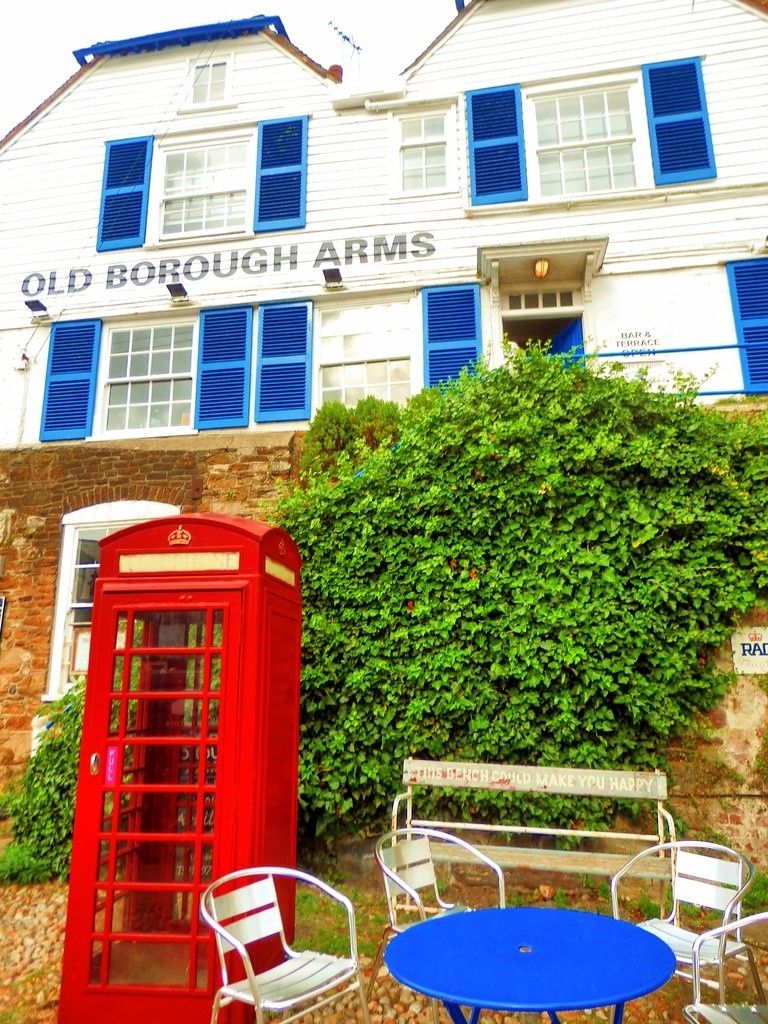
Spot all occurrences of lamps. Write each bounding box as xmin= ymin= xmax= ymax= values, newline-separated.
xmin=24 ymin=300 xmax=52 ymax=321
xmin=320 ymin=268 xmax=344 ymax=287
xmin=168 ymin=282 xmax=190 ymax=304
xmin=533 ymin=258 xmax=550 ymax=279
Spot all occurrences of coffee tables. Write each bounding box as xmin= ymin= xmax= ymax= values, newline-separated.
xmin=384 ymin=904 xmax=677 ymax=1024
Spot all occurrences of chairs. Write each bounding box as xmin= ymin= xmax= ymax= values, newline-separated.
xmin=611 ymin=841 xmax=766 ymax=1007
xmin=680 ymin=909 xmax=768 ymax=1024
xmin=360 ymin=827 xmax=506 ymax=1023
xmin=200 ymin=866 xmax=371 ymax=1024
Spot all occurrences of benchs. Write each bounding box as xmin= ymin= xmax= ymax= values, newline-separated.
xmin=388 ymin=758 xmax=680 ymax=927
